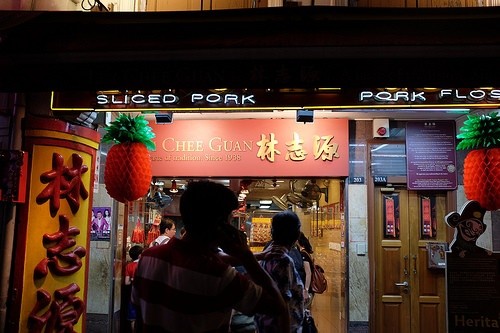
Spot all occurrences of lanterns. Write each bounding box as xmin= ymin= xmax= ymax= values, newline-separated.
xmin=99 ymin=112 xmax=157 ymax=204
xmin=455 ymin=112 xmax=499 ymax=209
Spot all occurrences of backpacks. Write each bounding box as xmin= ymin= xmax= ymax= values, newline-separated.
xmin=302 ymin=250 xmax=328 ymax=294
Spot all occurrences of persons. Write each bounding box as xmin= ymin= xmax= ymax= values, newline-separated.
xmin=253 ymin=209 xmax=319 ymax=333
xmin=216 ymin=245 xmax=285 ymax=274
xmin=125 ymin=245 xmax=143 ymax=331
xmin=179 ymin=227 xmax=187 ymax=239
xmin=91 ymin=208 xmax=112 ymax=240
xmin=148 ymin=217 xmax=177 ymax=248
xmin=130 ymin=178 xmax=286 ymax=333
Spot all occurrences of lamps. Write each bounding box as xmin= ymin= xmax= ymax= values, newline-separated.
xmin=169 ymin=179 xmax=178 ymax=193
xmin=155 ymin=112 xmax=173 ymax=125
xmin=296 ymin=110 xmax=314 ymax=124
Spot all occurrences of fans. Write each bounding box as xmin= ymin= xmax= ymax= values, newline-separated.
xmin=146 ymin=192 xmax=172 ymax=209
xmin=271 ymin=179 xmax=328 ymax=212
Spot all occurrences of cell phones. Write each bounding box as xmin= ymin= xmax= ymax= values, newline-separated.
xmin=218 ymin=223 xmax=246 ymax=247
